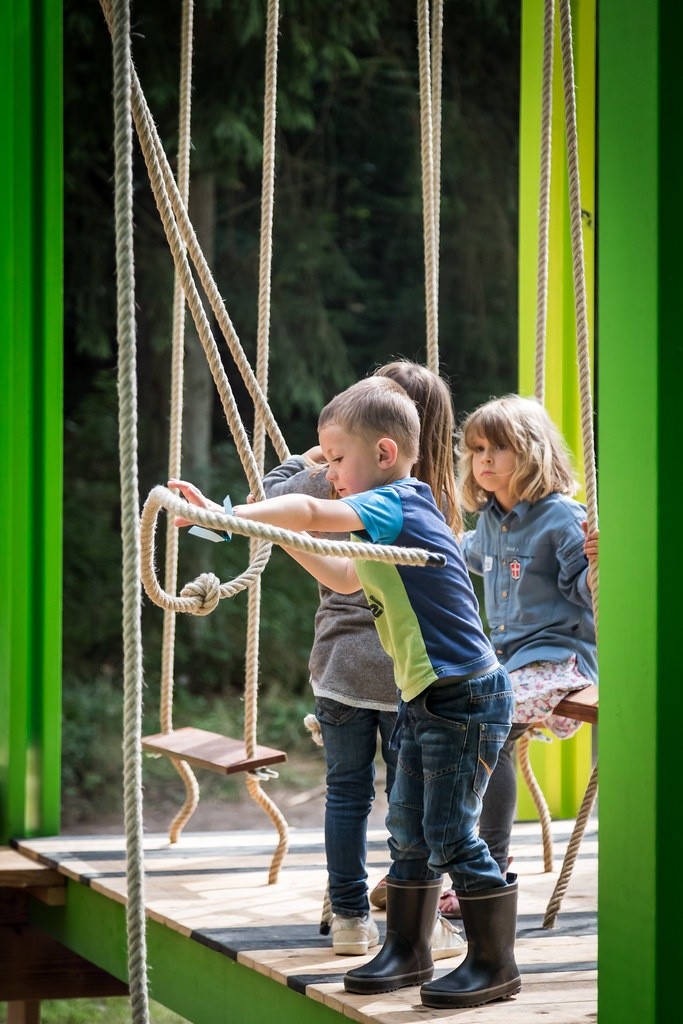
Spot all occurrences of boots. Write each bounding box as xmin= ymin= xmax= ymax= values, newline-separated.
xmin=344 ymin=874 xmax=444 ymax=994
xmin=420 ymin=872 xmax=521 ymax=1008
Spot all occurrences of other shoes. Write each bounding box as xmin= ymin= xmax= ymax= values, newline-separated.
xmin=370 ymin=877 xmax=387 ymax=908
xmin=437 ymin=889 xmax=463 ymax=919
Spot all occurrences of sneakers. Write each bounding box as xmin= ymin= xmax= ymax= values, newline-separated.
xmin=430 ymin=915 xmax=464 ymax=961
xmin=331 ymin=911 xmax=379 ymax=955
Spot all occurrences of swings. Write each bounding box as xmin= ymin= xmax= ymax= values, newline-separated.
xmin=103 ymin=0 xmax=599 ymax=1024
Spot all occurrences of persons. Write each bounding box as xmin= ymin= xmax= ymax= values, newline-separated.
xmin=261 ymin=361 xmax=464 ymax=961
xmin=167 ymin=377 xmax=521 ymax=1009
xmin=436 ymin=395 xmax=599 ymax=921
